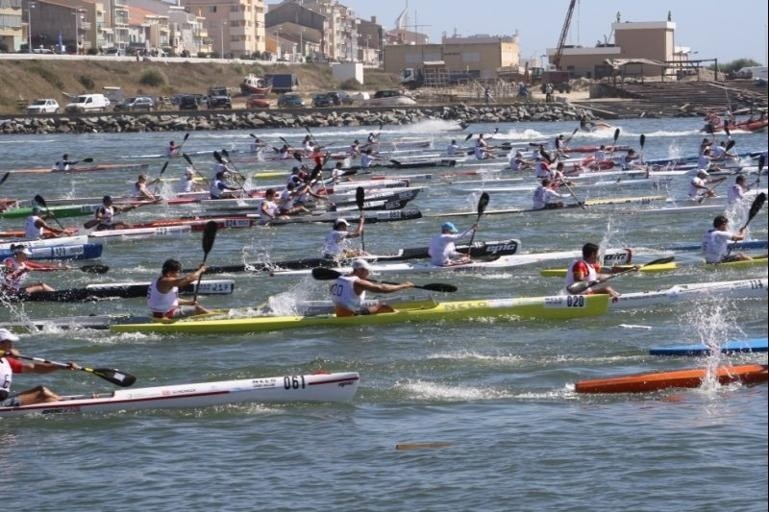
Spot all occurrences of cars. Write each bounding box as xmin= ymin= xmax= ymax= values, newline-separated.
xmin=28 ymin=42 xmax=169 ymax=59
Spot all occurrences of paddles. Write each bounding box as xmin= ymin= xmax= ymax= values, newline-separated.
xmin=6 ymin=351 xmax=136 ymax=386
xmin=695 ymin=126 xmax=768 ymax=260
xmin=355 ymin=184 xmax=366 ymax=251
xmin=454 ymin=126 xmax=644 ymax=166
xmin=193 ymin=221 xmax=218 ymax=300
xmin=312 ymin=268 xmax=457 ymax=292
xmin=85 ymin=206 xmax=132 ymax=229
xmin=568 ymin=256 xmax=675 ymax=295
xmin=152 ymin=125 xmax=400 ymax=197
xmin=34 ymin=195 xmax=65 ymax=234
xmin=71 ymin=157 xmax=95 ymax=164
xmin=24 ymin=263 xmax=108 ymax=274
xmin=468 ymin=192 xmax=489 ymax=255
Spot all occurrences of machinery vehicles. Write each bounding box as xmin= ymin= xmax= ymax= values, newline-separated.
xmin=540 ymin=0 xmax=581 ymax=93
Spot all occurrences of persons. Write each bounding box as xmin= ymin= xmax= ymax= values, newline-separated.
xmin=561 ymin=242 xmax=622 ymax=300
xmin=494 ymin=128 xmax=499 ymax=134
xmin=510 ymin=133 xmax=642 ymax=209
xmin=128 ymin=135 xmax=383 ymax=221
xmin=145 ymin=216 xmax=481 ymax=319
xmin=447 ymin=133 xmax=495 ymax=160
xmin=2 ymin=153 xmax=138 ymax=407
xmin=688 ymin=109 xmax=754 ymax=267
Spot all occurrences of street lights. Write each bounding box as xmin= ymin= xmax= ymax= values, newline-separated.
xmin=365 ymin=31 xmax=373 ymax=63
xmin=27 ymin=1 xmax=36 ymax=53
xmin=219 ymin=20 xmax=227 ymax=58
xmin=74 ymin=5 xmax=83 ymax=54
xmin=299 ymin=27 xmax=305 ymax=57
xmin=276 ymin=25 xmax=282 ymax=49
xmin=117 ymin=8 xmax=125 ymax=49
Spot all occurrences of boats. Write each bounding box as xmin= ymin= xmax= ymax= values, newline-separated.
xmin=645 ymin=338 xmax=769 ymax=356
xmin=0 ymin=369 xmax=361 ymax=424
xmin=239 ymin=72 xmax=273 ymax=96
xmin=574 ymin=359 xmax=769 ymax=394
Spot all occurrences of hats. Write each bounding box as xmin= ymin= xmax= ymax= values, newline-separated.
xmin=0 ymin=328 xmax=20 ymax=343
xmin=13 ymin=247 xmax=32 ymax=255
xmin=352 ymin=259 xmax=374 ymax=273
xmin=697 ymin=168 xmax=710 ymax=176
xmin=441 ymin=221 xmax=459 ymax=234
xmin=335 ymin=217 xmax=351 ymax=227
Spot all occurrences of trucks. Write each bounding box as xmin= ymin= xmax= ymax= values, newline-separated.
xmin=401 ymin=65 xmax=480 ymax=89
xmin=256 ymin=73 xmax=299 ymax=94
xmin=495 ymin=64 xmax=544 ymax=85
